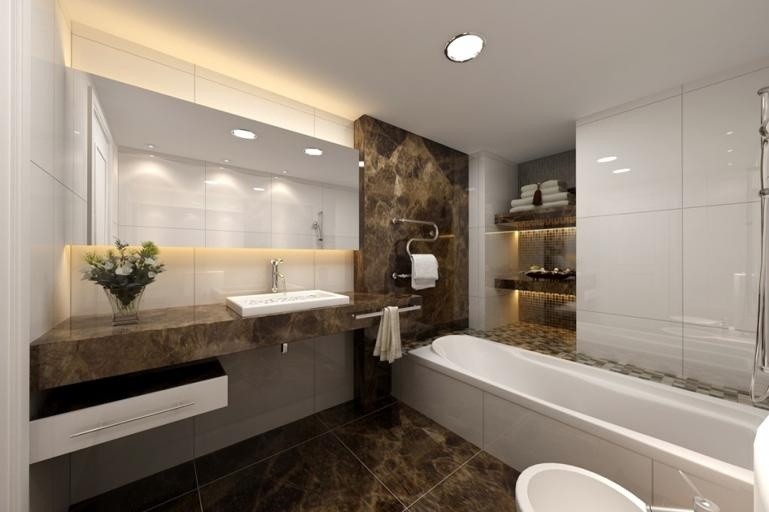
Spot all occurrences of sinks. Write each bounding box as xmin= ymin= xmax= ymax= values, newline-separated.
xmin=517 ymin=462 xmax=649 ymax=512
xmin=226 ymin=290 xmax=350 ymax=319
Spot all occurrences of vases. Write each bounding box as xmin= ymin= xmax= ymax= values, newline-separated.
xmin=107 ymin=285 xmax=143 ymax=323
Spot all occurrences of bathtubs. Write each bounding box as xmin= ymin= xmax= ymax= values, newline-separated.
xmin=410 ymin=332 xmax=768 ymax=497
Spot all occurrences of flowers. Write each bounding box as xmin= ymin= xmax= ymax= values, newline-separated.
xmin=78 ymin=244 xmax=161 ymax=315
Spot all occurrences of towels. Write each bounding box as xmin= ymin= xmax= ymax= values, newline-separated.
xmin=407 ymin=254 xmax=437 ymax=290
xmin=373 ymin=306 xmax=406 ymax=364
xmin=509 ymin=180 xmax=575 ymax=215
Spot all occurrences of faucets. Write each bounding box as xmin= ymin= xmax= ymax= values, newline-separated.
xmin=272 ymin=258 xmax=284 ymax=291
xmin=646 ymin=468 xmax=721 ymax=512
